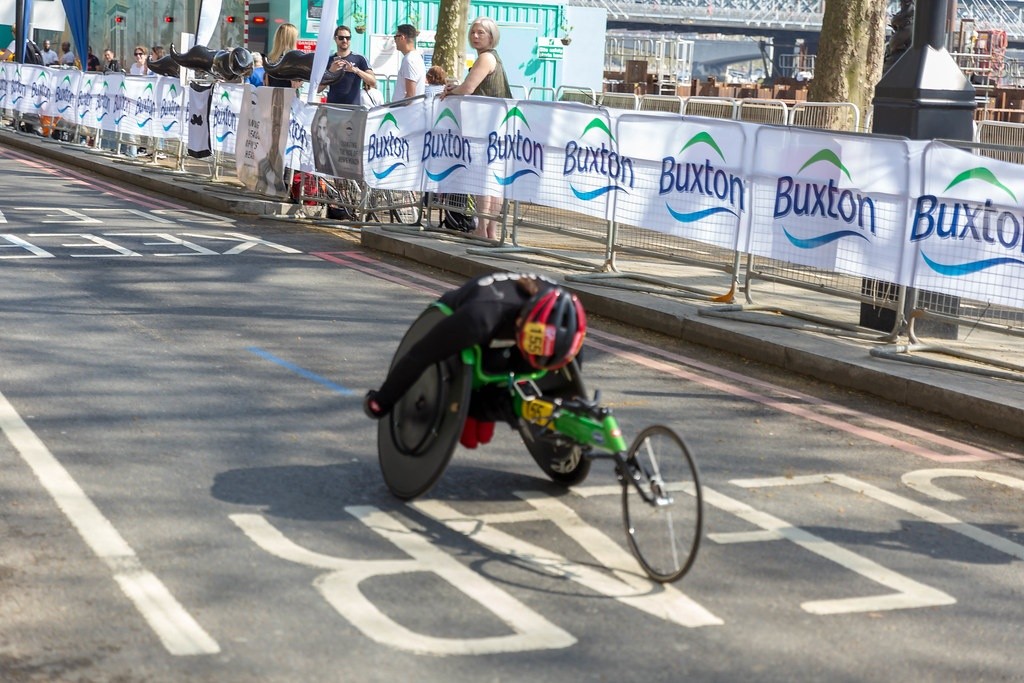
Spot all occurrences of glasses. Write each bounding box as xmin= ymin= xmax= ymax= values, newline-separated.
xmin=134 ymin=53 xmax=143 ymax=56
xmin=336 ymin=36 xmax=351 ymax=40
xmin=394 ymin=35 xmax=406 ymax=40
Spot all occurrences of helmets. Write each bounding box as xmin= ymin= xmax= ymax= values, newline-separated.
xmin=515 ymin=286 xmax=587 ymax=371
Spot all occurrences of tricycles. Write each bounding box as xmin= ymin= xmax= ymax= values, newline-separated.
xmin=377 ymin=297 xmax=704 ymax=585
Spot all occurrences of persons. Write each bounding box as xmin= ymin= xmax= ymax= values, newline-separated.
xmin=439 ymin=17 xmax=513 ymax=236
xmin=317 ymin=25 xmax=377 ymax=104
xmin=244 ymin=51 xmax=266 ymax=86
xmin=265 ymin=23 xmax=304 ymax=97
xmin=361 ymin=80 xmax=384 ymax=109
xmin=60 ymin=41 xmax=74 ymax=66
xmin=40 ymin=39 xmax=59 ymax=66
xmin=144 ymin=46 xmax=166 ymax=159
xmin=0 ymin=23 xmax=36 ymax=63
xmin=102 ymin=48 xmax=121 ymax=72
xmin=385 ymin=25 xmax=426 ymax=213
xmin=87 ymin=47 xmax=100 ymax=71
xmin=425 ymin=66 xmax=447 ymax=97
xmin=362 ymin=272 xmax=588 ymax=449
xmin=129 ymin=45 xmax=150 ymax=155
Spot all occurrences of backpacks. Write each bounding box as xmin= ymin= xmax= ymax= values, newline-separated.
xmin=26 ymin=40 xmax=44 ymax=66
xmin=438 ymin=193 xmax=471 ymax=233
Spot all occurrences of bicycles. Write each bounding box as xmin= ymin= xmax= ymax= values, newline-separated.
xmin=280 ymin=166 xmax=482 ymax=230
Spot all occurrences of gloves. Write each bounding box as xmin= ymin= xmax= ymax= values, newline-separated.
xmin=363 ymin=389 xmax=389 ymax=419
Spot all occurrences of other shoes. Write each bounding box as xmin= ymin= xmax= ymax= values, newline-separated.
xmin=478 ymin=421 xmax=494 ymax=444
xmin=384 ymin=200 xmax=418 ymax=215
xmin=469 ymin=229 xmax=496 ymax=241
xmin=459 ymin=417 xmax=479 ymax=448
xmin=137 ymin=147 xmax=148 ymax=157
xmin=146 ymin=151 xmax=167 ymax=160
